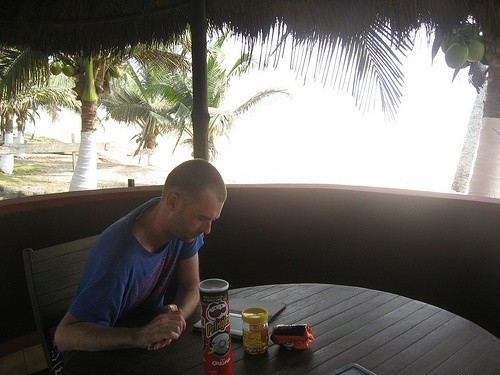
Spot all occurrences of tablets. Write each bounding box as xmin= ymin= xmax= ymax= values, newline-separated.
xmin=193 ymin=313 xmax=243 ymax=339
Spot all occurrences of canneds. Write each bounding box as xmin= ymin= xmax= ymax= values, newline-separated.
xmin=242 ymin=307 xmax=269 ymax=357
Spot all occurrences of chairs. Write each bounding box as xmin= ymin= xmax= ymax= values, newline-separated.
xmin=23 ymin=235 xmax=101 ymax=375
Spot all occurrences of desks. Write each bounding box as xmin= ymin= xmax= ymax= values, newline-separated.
xmin=64 ymin=283 xmax=500 ymax=375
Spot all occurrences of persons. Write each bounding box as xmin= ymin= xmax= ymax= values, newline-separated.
xmin=49 ymin=159 xmax=228 ymax=375
